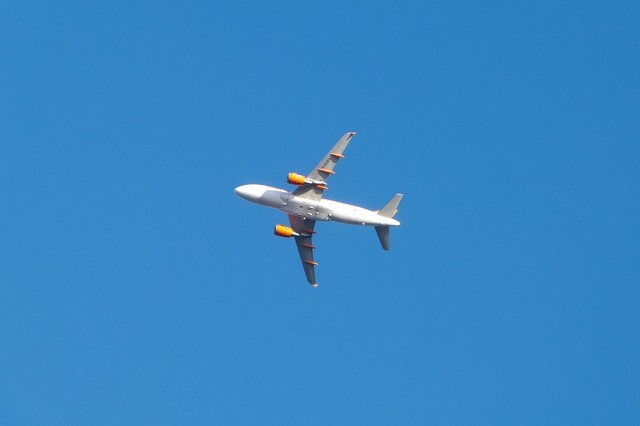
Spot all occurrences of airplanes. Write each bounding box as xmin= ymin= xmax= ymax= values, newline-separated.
xmin=234 ymin=132 xmax=403 ymax=287
